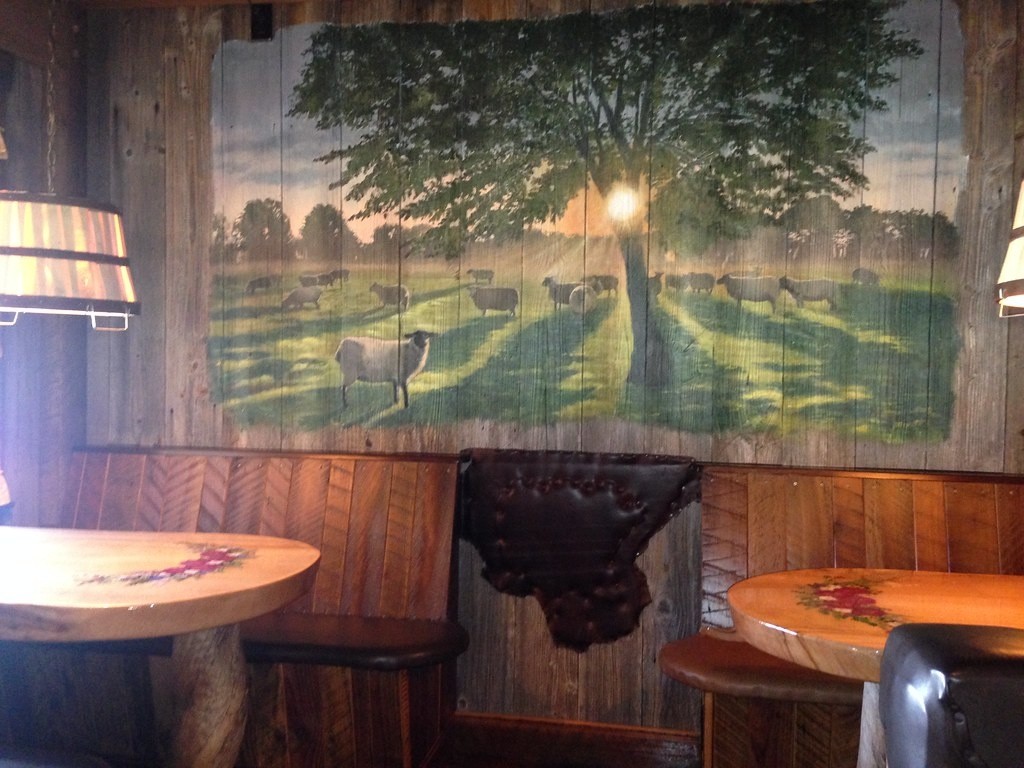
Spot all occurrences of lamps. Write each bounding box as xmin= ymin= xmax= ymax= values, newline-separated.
xmin=1 ymin=189 xmax=140 ymax=331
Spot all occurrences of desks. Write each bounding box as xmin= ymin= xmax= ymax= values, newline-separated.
xmin=0 ymin=525 xmax=321 ymax=768
xmin=724 ymin=566 xmax=1024 ymax=768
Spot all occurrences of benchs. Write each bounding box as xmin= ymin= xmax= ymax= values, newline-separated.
xmin=19 ymin=446 xmax=1024 ymax=768
